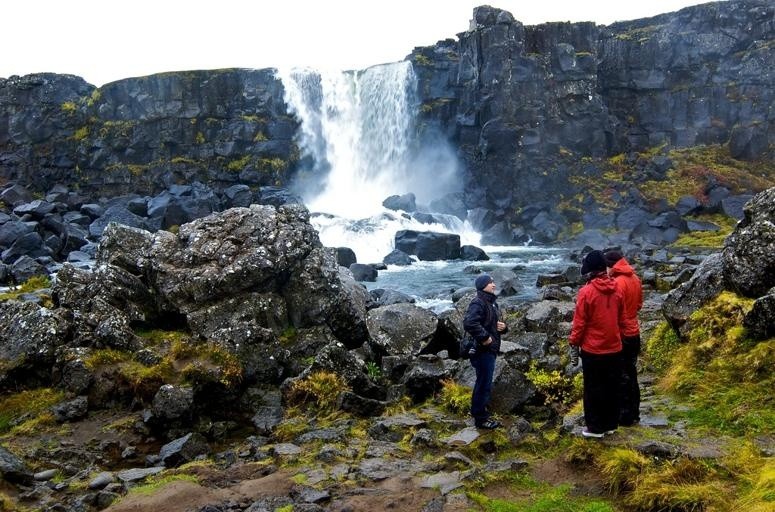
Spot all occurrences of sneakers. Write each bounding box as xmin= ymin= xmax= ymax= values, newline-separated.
xmin=606 ymin=427 xmax=620 ymax=435
xmin=581 ymin=428 xmax=606 ymax=439
xmin=474 ymin=419 xmax=501 ymax=430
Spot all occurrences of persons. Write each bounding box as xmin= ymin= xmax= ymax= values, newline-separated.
xmin=566 ymin=247 xmax=624 ymax=440
xmin=464 ymin=274 xmax=509 ymax=430
xmin=603 ymin=252 xmax=644 ymax=423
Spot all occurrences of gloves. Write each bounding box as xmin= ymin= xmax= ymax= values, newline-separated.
xmin=569 ymin=344 xmax=579 ymax=367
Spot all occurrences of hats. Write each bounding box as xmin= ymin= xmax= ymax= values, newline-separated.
xmin=604 ymin=249 xmax=624 ymax=269
xmin=474 ymin=275 xmax=495 ymax=291
xmin=579 ymin=249 xmax=607 ymax=275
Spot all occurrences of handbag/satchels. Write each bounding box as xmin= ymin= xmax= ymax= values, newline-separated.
xmin=459 ymin=327 xmax=481 ymax=361
